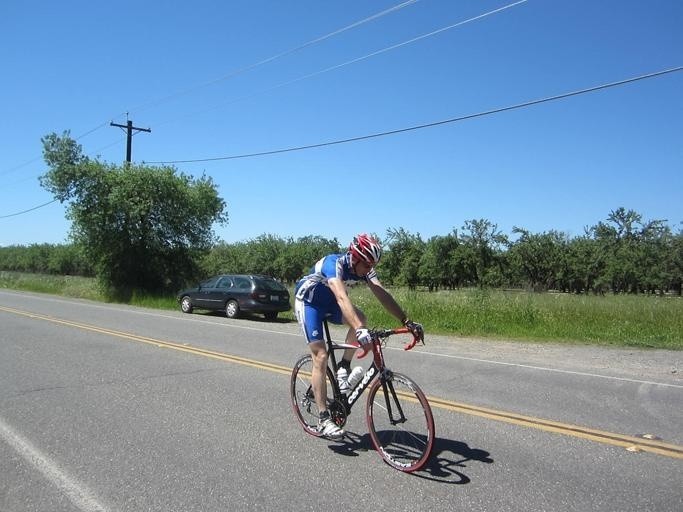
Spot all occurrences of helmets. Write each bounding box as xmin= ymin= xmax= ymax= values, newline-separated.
xmin=348 ymin=234 xmax=382 ymax=263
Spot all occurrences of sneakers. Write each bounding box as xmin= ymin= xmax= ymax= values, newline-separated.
xmin=316 ymin=416 xmax=346 ymax=437
xmin=334 ymin=364 xmax=352 ymax=382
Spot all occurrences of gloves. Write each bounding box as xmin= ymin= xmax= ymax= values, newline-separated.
xmin=356 ymin=328 xmax=373 ymax=345
xmin=404 ymin=320 xmax=424 ymax=339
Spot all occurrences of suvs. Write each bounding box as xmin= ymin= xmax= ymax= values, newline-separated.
xmin=174 ymin=271 xmax=291 ymax=321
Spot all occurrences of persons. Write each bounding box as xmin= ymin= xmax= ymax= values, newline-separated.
xmin=293 ymin=231 xmax=426 ymax=440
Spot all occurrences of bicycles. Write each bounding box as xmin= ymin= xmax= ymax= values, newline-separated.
xmin=286 ymin=305 xmax=437 ymax=477
